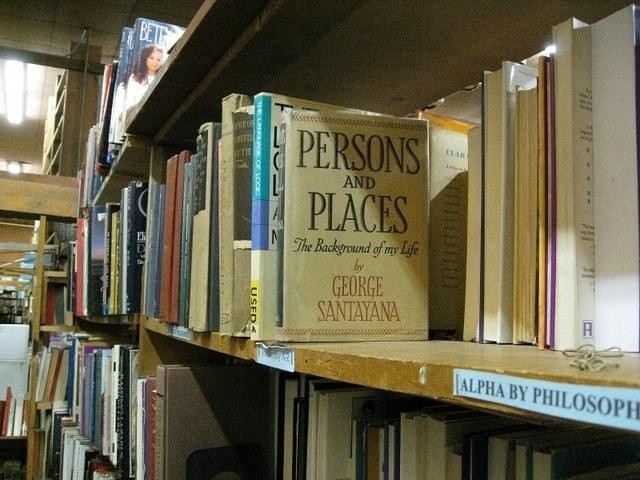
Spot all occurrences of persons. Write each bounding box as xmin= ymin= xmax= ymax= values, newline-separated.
xmin=129 ymin=44 xmax=164 ymax=105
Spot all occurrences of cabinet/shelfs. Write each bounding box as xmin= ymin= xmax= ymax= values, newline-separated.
xmin=42 ymin=43 xmax=102 ymax=177
xmin=0 ymin=290 xmax=31 ymax=323
xmin=65 ymin=135 xmax=152 ymax=326
xmin=125 ymin=0 xmax=640 ymax=432
xmin=27 ymin=216 xmax=79 ymax=478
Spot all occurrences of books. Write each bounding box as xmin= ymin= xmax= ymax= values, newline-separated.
xmin=80 ymin=16 xmax=188 ymax=209
xmin=134 ymin=359 xmax=636 ymax=480
xmin=144 ymin=7 xmax=637 ymax=344
xmin=73 ymin=179 xmax=148 ymax=314
xmin=0 ymin=332 xmax=139 ymax=480
